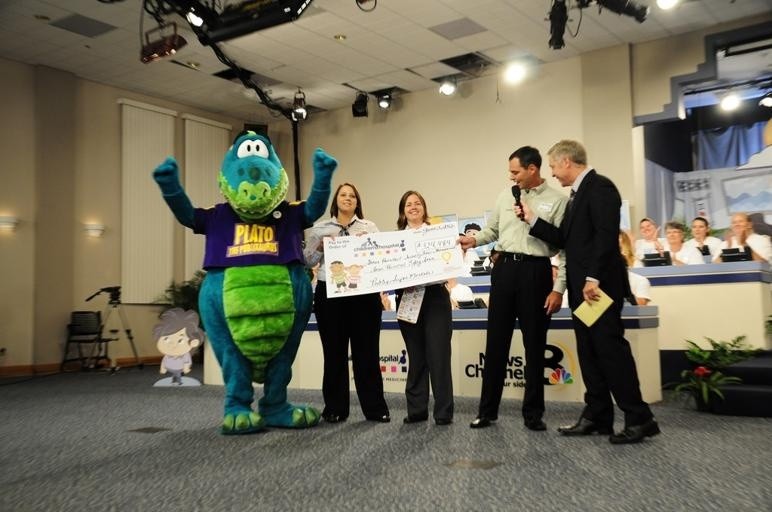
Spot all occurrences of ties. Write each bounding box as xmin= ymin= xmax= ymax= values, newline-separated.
xmin=569 ymin=189 xmax=577 ymax=210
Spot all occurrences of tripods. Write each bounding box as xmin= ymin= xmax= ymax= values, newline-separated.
xmin=85 ymin=286 xmax=141 ymax=369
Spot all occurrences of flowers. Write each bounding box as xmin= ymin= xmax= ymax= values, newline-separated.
xmin=669 ymin=367 xmax=742 ymax=403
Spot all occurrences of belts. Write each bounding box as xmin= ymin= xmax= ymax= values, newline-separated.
xmin=502 ymin=253 xmax=534 ymax=261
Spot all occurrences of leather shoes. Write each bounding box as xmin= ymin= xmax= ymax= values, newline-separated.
xmin=366 ymin=414 xmax=391 ymax=423
xmin=470 ymin=417 xmax=498 ymax=429
xmin=325 ymin=414 xmax=340 ymax=423
xmin=558 ymin=418 xmax=615 ymax=436
xmin=436 ymin=420 xmax=451 ymax=425
xmin=524 ymin=420 xmax=547 ymax=431
xmin=609 ymin=417 xmax=662 ymax=445
xmin=403 ymin=417 xmax=415 ymax=424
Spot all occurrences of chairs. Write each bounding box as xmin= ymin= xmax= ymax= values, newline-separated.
xmin=61 ymin=311 xmax=118 ymax=373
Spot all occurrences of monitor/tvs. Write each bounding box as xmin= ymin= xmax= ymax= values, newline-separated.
xmin=189 ymin=0 xmax=339 ymax=45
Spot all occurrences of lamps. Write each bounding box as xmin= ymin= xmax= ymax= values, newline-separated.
xmin=291 ymin=97 xmax=308 ymax=122
xmin=758 ymin=90 xmax=772 ymax=110
xmin=378 ymin=91 xmax=392 ymax=110
xmin=440 ymin=74 xmax=457 ymax=98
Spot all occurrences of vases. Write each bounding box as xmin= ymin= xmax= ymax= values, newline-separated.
xmin=694 ymin=394 xmax=710 ymax=412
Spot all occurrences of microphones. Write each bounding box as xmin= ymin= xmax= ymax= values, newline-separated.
xmin=512 ymin=185 xmax=524 ymax=221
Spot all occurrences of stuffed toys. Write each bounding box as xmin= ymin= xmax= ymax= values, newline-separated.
xmin=150 ymin=127 xmax=339 ymax=438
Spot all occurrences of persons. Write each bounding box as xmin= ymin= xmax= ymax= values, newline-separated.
xmin=618 ymin=212 xmax=771 ymax=305
xmin=300 ymin=181 xmax=393 ymax=425
xmin=454 ymin=144 xmax=567 ymax=434
xmin=447 ymin=277 xmax=476 ymax=308
xmin=390 ymin=188 xmax=455 ymax=429
xmin=513 ymin=139 xmax=663 ymax=447
